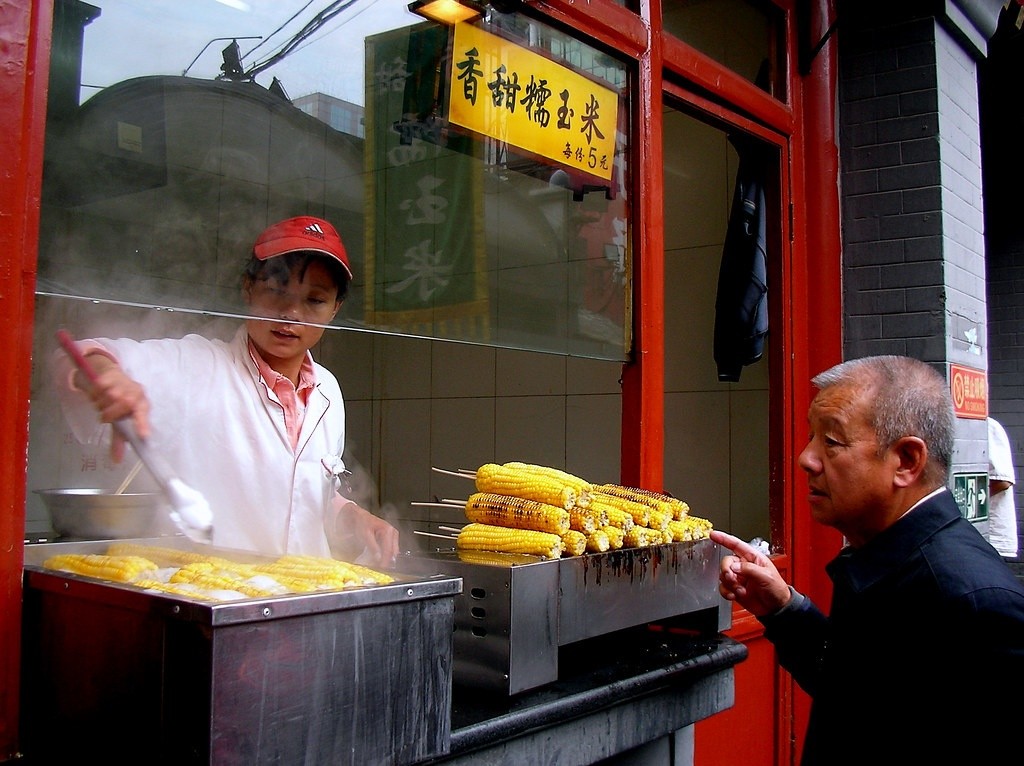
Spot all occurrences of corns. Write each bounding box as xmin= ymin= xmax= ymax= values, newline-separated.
xmin=42 ymin=543 xmax=396 ymax=604
xmin=455 ymin=550 xmax=542 ymax=566
xmin=455 ymin=461 xmax=712 ymax=560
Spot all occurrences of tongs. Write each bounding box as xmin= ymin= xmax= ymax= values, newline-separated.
xmin=54 ymin=329 xmax=215 ymax=544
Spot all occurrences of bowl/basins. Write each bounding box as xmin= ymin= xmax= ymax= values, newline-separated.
xmin=31 ymin=489 xmax=168 ymax=538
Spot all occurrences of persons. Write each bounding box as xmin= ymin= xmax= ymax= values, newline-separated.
xmin=986 ymin=415 xmax=1019 ymax=559
xmin=709 ymin=353 xmax=1024 ymax=766
xmin=45 ymin=214 xmax=401 ymax=568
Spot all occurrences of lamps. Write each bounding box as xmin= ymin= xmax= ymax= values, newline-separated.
xmin=406 ymin=0 xmax=487 ymax=26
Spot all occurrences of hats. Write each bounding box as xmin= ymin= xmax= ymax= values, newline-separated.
xmin=252 ymin=216 xmax=352 ymax=280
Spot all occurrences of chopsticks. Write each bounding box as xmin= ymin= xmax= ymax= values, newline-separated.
xmin=116 ymin=460 xmax=143 ymax=495
